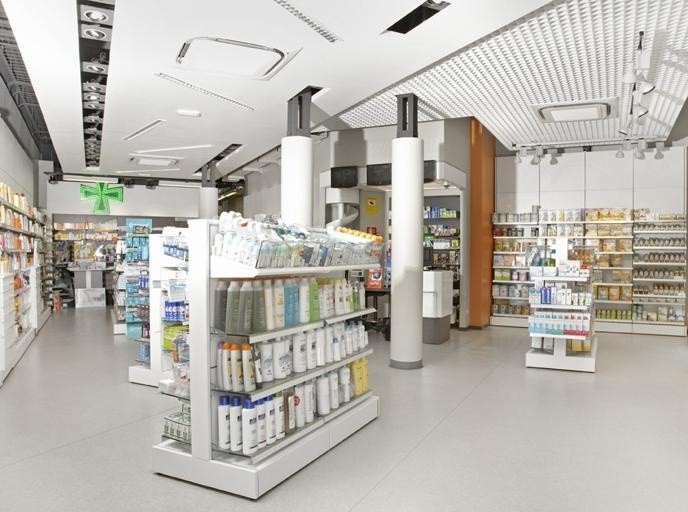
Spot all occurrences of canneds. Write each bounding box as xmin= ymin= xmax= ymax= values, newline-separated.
xmin=493 ymin=227 xmax=538 ymax=236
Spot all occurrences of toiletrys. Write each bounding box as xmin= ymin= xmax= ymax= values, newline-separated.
xmin=214 ymin=277 xmax=368 ymax=393
xmin=527 ymin=311 xmax=591 ymax=338
xmin=528 ymin=286 xmax=557 ymax=305
xmin=316 ymin=356 xmax=369 ymax=416
xmin=557 ymin=289 xmax=592 ymax=306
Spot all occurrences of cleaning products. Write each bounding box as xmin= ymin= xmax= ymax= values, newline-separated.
xmin=217 ymin=379 xmax=316 ymax=456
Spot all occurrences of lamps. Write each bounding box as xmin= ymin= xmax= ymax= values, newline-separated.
xmin=508 ymin=135 xmax=668 ymax=167
xmin=618 ymin=29 xmax=655 ymax=142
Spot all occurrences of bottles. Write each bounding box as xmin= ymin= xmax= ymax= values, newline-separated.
xmin=594 ymin=225 xmax=686 ymax=323
xmin=214 ymin=272 xmax=369 ymax=436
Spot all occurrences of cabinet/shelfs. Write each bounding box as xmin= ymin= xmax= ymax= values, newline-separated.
xmin=2 ymin=178 xmax=105 ymax=395
xmin=492 ymin=205 xmax=688 ymax=338
xmin=104 ymin=215 xmax=188 ymax=387
xmin=152 ymin=215 xmax=386 ymax=503
xmin=524 ymin=238 xmax=598 ymax=373
xmin=424 ymin=194 xmax=471 ymax=331
